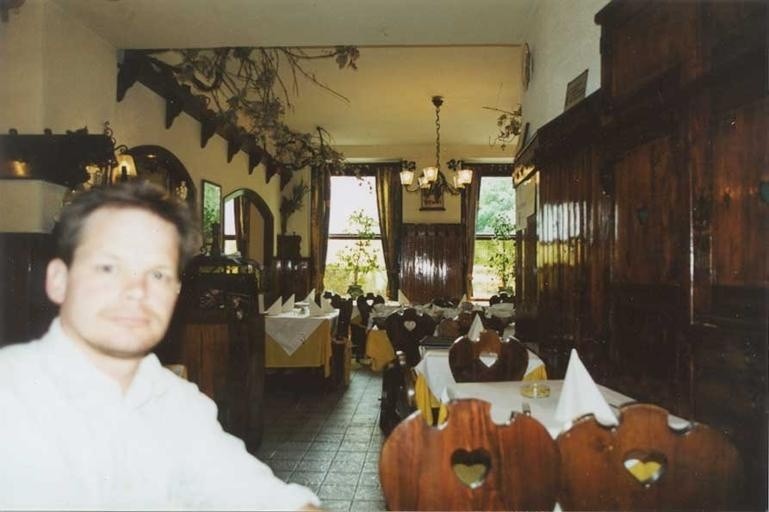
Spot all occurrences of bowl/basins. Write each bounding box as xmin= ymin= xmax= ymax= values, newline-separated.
xmin=1 ymin=160 xmax=32 ymax=178
xmin=520 ymin=384 xmax=551 ymax=397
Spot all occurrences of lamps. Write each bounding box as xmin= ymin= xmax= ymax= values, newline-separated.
xmin=63 ymin=119 xmax=138 ymax=205
xmin=398 ymin=95 xmax=473 ymax=201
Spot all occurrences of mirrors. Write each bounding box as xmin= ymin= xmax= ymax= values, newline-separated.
xmin=200 ymin=180 xmax=223 ymax=254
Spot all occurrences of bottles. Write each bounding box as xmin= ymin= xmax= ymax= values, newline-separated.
xmin=175 ymin=180 xmax=189 ymax=201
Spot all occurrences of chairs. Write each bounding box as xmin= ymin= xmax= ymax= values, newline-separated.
xmin=314 ymin=292 xmax=521 ymax=384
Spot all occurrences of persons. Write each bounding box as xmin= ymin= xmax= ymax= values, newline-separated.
xmin=0 ymin=173 xmax=332 ymax=511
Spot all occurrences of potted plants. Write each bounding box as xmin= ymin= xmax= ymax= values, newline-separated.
xmin=335 ymin=207 xmax=380 ymax=298
xmin=485 ymin=212 xmax=517 ymax=295
xmin=276 ymin=175 xmax=311 ymax=266
